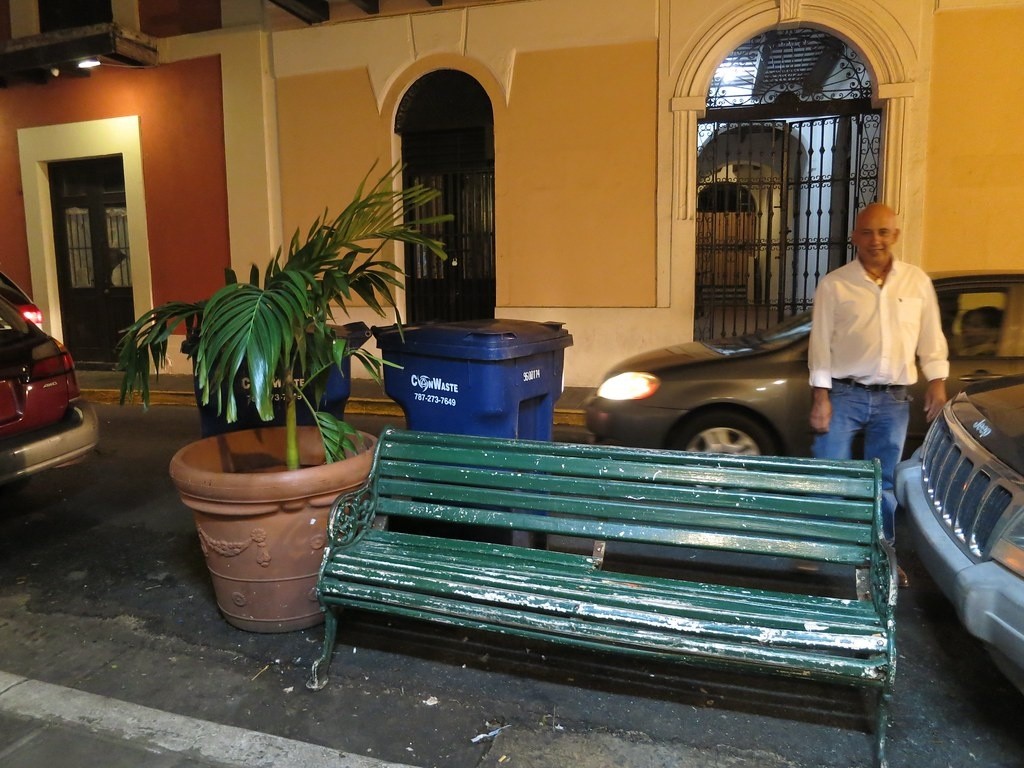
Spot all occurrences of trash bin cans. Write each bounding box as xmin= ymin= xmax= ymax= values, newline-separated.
xmin=180 ymin=319 xmax=376 ymax=434
xmin=372 ymin=320 xmax=574 ymax=550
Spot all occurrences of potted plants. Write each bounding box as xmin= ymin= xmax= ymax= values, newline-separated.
xmin=108 ymin=156 xmax=454 ymax=633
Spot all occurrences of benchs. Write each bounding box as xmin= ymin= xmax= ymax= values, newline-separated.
xmin=308 ymin=426 xmax=897 ymax=768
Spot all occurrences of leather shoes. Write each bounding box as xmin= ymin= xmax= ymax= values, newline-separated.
xmin=896 ymin=566 xmax=910 ymax=589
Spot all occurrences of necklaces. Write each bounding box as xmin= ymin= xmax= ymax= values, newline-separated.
xmin=865 ymin=259 xmax=890 ymax=285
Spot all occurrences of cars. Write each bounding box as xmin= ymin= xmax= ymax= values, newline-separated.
xmin=585 ymin=266 xmax=1024 ymax=492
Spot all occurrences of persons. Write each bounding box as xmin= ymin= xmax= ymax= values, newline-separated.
xmin=807 ymin=204 xmax=949 ymax=601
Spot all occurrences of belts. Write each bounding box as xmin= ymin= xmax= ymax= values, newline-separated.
xmin=831 ymin=377 xmax=905 ymax=392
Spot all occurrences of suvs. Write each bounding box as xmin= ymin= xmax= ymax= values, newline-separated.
xmin=893 ymin=373 xmax=1024 ymax=694
xmin=0 ymin=272 xmax=99 ymax=486
